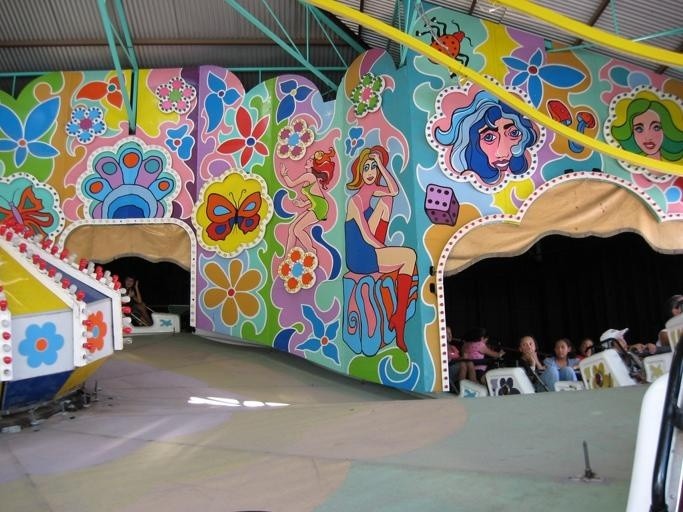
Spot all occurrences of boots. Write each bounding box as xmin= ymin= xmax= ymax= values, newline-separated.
xmin=388 ymin=273 xmax=412 ymax=352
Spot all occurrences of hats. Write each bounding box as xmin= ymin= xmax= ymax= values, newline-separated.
xmin=600 ymin=327 xmax=629 ymax=348
xmin=667 ymin=295 xmax=683 ymax=307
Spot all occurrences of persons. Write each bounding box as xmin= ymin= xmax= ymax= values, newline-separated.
xmin=444 ymin=320 xmax=664 ymax=394
xmin=120 ymin=273 xmax=153 ymax=326
xmin=665 ymin=293 xmax=682 ymax=330
